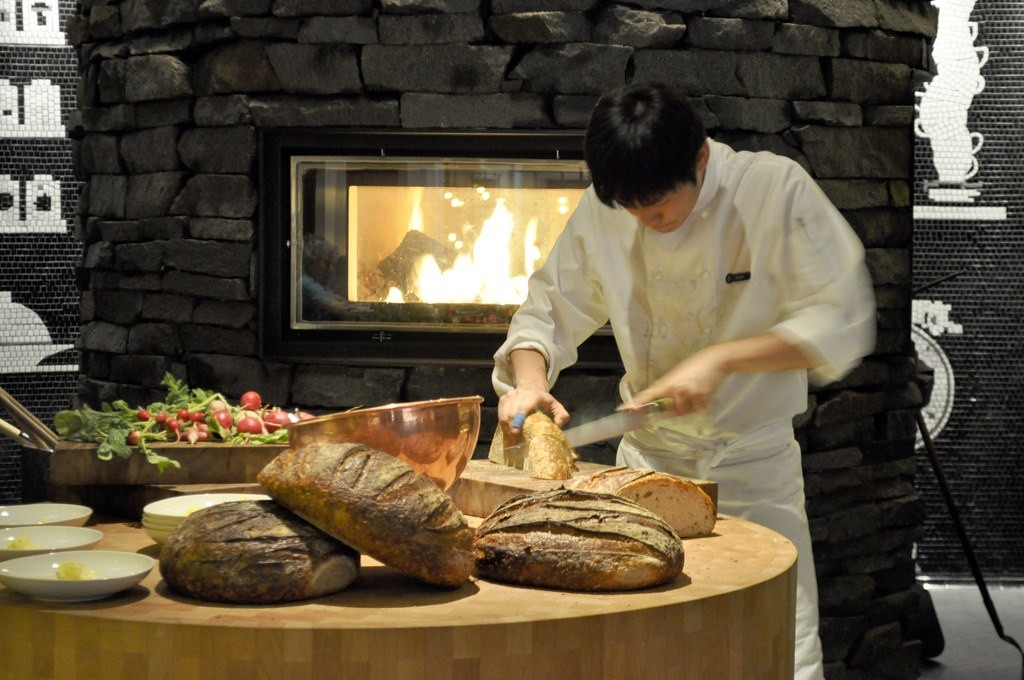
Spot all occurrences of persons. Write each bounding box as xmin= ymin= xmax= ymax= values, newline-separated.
xmin=485 ymin=80 xmax=877 ymax=680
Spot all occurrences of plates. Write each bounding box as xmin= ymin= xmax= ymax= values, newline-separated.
xmin=1 ymin=551 xmax=154 ymax=603
xmin=1 ymin=526 xmax=103 ymax=561
xmin=141 ymin=493 xmax=275 ymax=549
xmin=1 ymin=502 xmax=93 ymax=531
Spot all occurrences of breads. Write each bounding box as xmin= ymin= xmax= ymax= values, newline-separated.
xmin=160 ymin=501 xmax=362 ymax=602
xmin=258 ymin=442 xmax=476 ymax=588
xmin=490 ymin=411 xmax=578 ymax=480
xmin=572 ymin=467 xmax=717 ymax=536
xmin=472 ymin=490 xmax=684 ymax=591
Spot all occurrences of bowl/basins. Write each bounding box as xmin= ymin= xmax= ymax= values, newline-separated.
xmin=281 ymin=395 xmax=485 ymax=492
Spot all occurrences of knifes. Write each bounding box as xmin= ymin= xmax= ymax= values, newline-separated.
xmin=500 ymin=397 xmax=682 ymax=457
